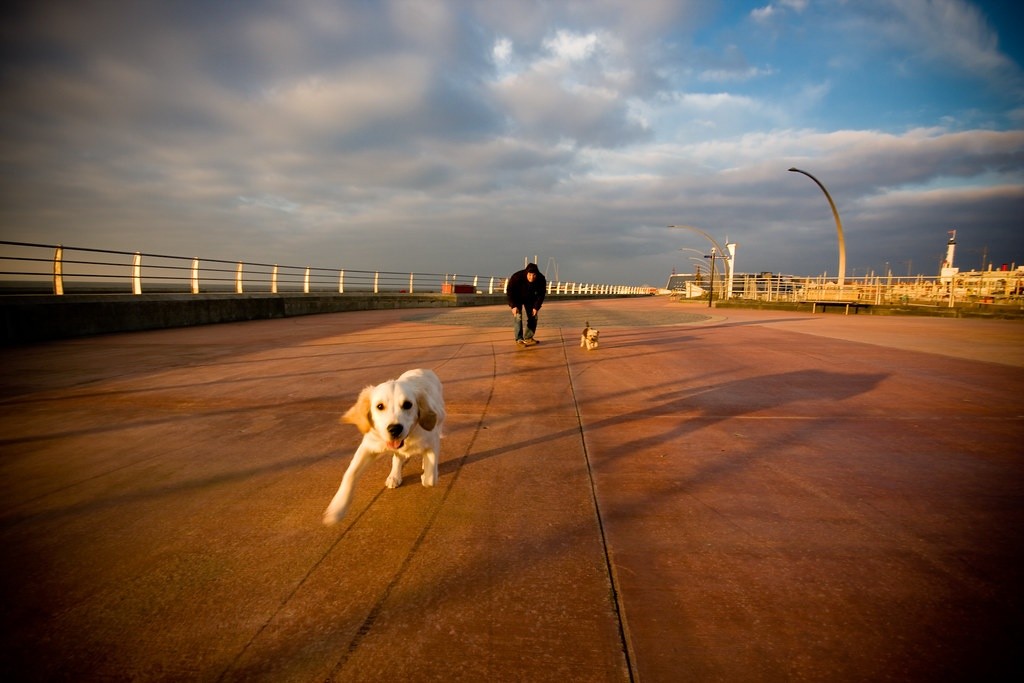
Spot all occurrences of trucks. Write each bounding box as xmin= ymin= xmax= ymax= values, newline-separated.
xmin=440 ymin=282 xmax=483 ymax=296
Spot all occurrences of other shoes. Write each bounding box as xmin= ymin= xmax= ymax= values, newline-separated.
xmin=516 ymin=341 xmax=526 ymax=348
xmin=525 ymin=337 xmax=538 ymax=344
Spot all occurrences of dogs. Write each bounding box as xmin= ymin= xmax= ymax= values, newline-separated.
xmin=580 ymin=320 xmax=600 ymax=352
xmin=321 ymin=367 xmax=446 ymax=528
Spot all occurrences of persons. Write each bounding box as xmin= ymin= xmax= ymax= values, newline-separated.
xmin=507 ymin=263 xmax=546 ymax=348
